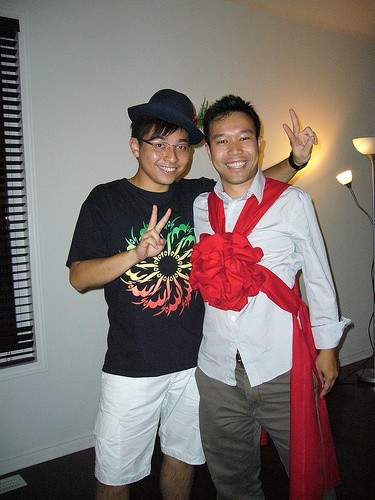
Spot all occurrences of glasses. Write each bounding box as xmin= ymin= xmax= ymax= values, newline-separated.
xmin=131 ymin=135 xmax=194 ymax=152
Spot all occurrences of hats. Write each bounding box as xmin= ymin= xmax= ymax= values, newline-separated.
xmin=127 ymin=88 xmax=206 ymax=145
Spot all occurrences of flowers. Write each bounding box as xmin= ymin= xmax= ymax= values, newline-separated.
xmin=189 ymin=232 xmax=264 ymax=312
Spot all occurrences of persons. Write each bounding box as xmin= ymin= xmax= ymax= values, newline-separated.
xmin=192 ymin=94 xmax=352 ymax=500
xmin=64 ymin=89 xmax=318 ymax=500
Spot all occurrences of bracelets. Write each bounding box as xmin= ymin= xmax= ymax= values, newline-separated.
xmin=288 ymin=153 xmax=308 ymax=171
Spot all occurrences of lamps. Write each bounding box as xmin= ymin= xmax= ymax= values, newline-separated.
xmin=335 ymin=137 xmax=375 ymax=383
